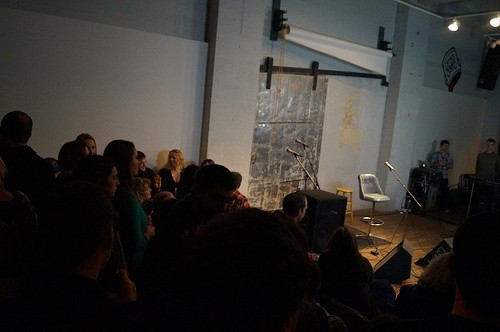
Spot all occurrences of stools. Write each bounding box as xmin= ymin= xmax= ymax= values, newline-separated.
xmin=354 ymin=174 xmax=390 ymax=245
xmin=336 ymin=188 xmax=354 ymax=223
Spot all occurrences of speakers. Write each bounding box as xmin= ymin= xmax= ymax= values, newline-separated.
xmin=373 ymin=239 xmax=412 ymax=284
xmin=296 ymin=189 xmax=347 ymax=255
xmin=477 ymin=39 xmax=500 ymax=91
xmin=414 ymin=237 xmax=455 ymax=266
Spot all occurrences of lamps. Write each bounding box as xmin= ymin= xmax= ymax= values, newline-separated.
xmin=447 ymin=18 xmax=461 ymax=32
xmin=489 ymin=14 xmax=500 ymax=28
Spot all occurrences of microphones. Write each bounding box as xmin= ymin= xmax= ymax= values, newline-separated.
xmin=286 ymin=147 xmax=304 ymax=157
xmin=295 ymin=139 xmax=310 ymax=148
xmin=385 ymin=161 xmax=395 ymax=171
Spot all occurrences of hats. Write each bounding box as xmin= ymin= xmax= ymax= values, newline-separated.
xmin=192 ymin=164 xmax=242 ymax=192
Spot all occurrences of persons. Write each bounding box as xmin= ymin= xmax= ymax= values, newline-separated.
xmin=0 ymin=110 xmax=500 ymax=332
xmin=429 ymin=139 xmax=453 ymax=213
xmin=476 ymin=137 xmax=500 ymax=178
